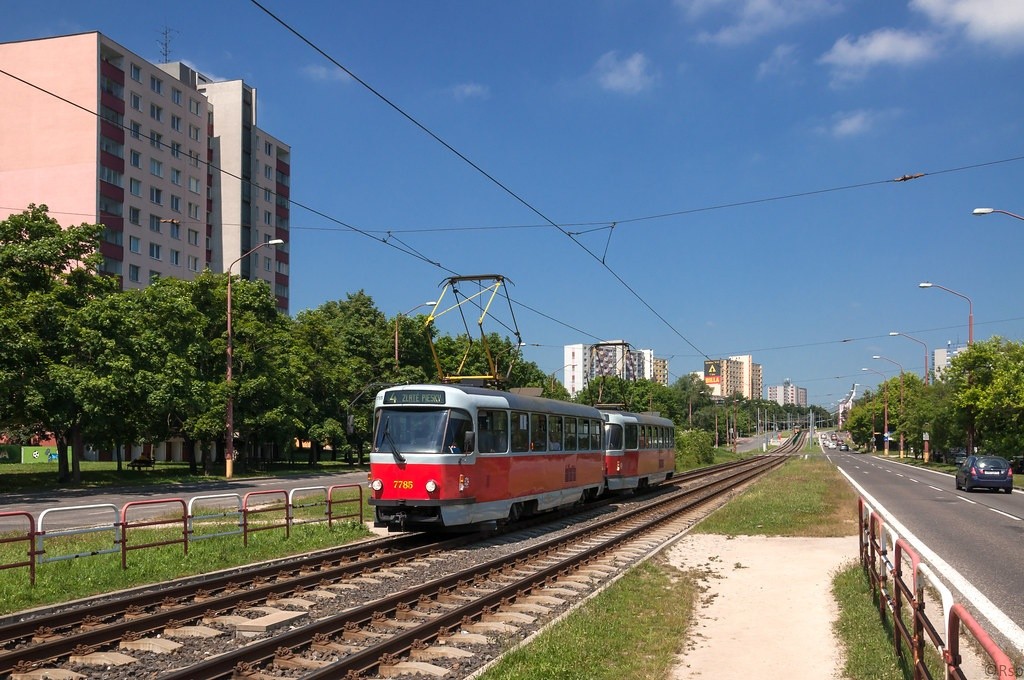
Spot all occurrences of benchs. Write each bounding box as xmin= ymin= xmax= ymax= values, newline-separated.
xmin=127 ymin=458 xmax=155 ymax=471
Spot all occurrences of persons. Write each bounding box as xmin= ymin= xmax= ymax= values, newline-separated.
xmin=937 ymin=452 xmax=943 ymax=463
xmin=551 ymin=434 xmax=560 ymax=451
xmin=137 ymin=453 xmax=151 ymax=471
xmin=567 ymin=434 xmax=598 ymax=450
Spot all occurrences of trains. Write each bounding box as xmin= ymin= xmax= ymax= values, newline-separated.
xmin=368 ymin=384 xmax=676 ymax=532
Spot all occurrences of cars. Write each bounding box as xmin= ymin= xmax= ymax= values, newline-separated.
xmin=837 ymin=440 xmax=844 ymax=446
xmin=823 ymin=439 xmax=829 ymax=444
xmin=1010 ymin=456 xmax=1024 ymax=474
xmin=955 ymin=455 xmax=1013 ymax=493
xmin=840 ymin=444 xmax=848 ymax=451
xmin=824 ymin=441 xmax=830 ymax=447
xmin=832 ymin=436 xmax=837 ymax=440
xmin=828 ymin=443 xmax=836 ymax=449
xmin=935 ymin=453 xmax=967 ymax=467
xmin=822 ymin=434 xmax=827 ymax=439
xmin=831 ymin=434 xmax=836 ymax=437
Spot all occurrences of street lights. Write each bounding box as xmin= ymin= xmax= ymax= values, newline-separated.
xmin=873 ymin=355 xmax=904 ymax=458
xmin=919 ymin=282 xmax=973 ymax=458
xmin=835 ymin=384 xmax=876 ymax=452
xmin=862 ymin=367 xmax=890 ymax=457
xmin=225 ymin=238 xmax=284 ymax=477
xmin=889 ymin=332 xmax=930 ymax=462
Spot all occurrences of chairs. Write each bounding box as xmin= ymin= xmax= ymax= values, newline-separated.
xmin=479 ymin=431 xmax=598 ymax=452
